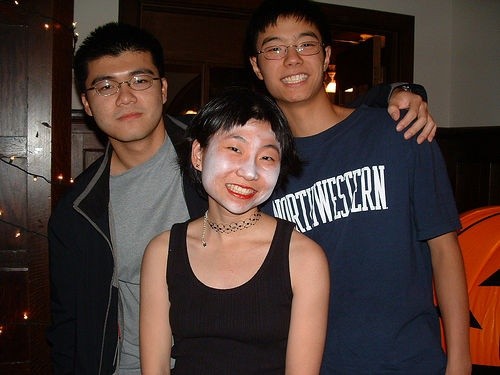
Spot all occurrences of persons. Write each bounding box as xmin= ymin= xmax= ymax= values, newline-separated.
xmin=46 ymin=22 xmax=437 ymax=375
xmin=243 ymin=0 xmax=472 ymax=375
xmin=139 ymin=90 xmax=330 ymax=375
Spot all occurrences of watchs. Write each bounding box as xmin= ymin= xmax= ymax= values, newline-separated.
xmin=400 ymin=85 xmax=411 ymax=92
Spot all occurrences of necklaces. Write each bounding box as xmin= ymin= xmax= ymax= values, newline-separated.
xmin=202 ymin=210 xmax=261 ymax=247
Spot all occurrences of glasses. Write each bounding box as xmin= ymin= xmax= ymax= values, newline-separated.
xmin=86 ymin=73 xmax=162 ymax=96
xmin=257 ymin=40 xmax=326 ymax=60
